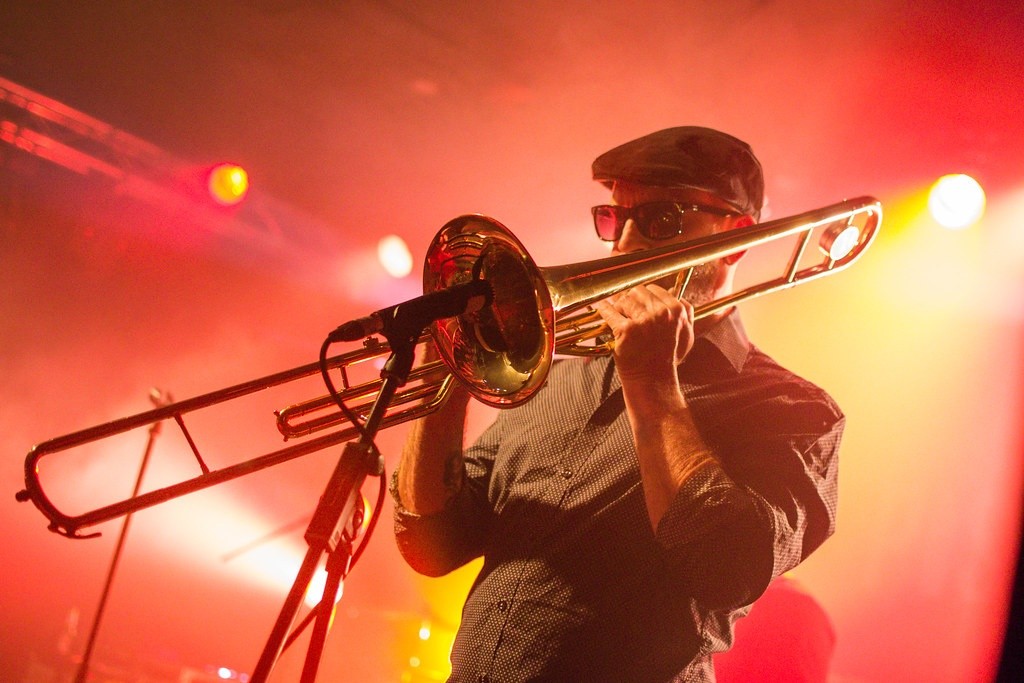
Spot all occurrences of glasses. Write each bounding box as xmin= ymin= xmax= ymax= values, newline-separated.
xmin=591 ymin=200 xmax=743 ymax=241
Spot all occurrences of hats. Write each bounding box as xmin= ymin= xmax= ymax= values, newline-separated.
xmin=592 ymin=126 xmax=765 ymax=224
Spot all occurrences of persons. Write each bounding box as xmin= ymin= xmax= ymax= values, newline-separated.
xmin=388 ymin=127 xmax=845 ymax=682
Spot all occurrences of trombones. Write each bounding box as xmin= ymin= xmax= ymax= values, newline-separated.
xmin=14 ymin=194 xmax=885 ymax=540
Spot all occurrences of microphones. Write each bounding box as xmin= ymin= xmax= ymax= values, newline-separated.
xmin=329 ymin=278 xmax=495 ymax=343
